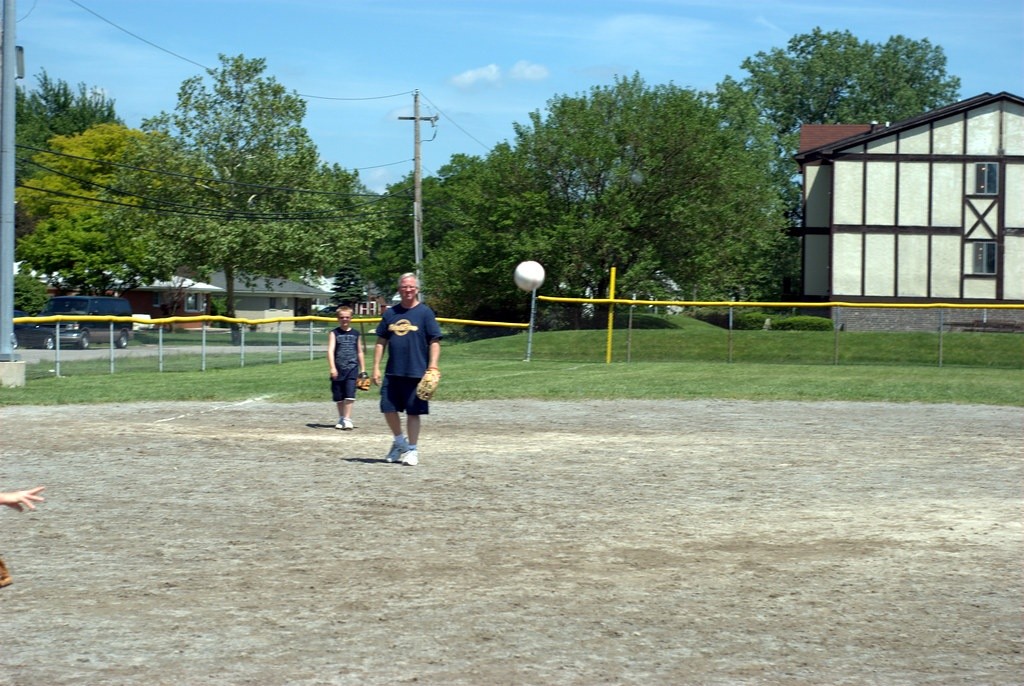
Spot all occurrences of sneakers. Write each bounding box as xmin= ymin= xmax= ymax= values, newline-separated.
xmin=386 ymin=438 xmax=408 ymax=463
xmin=401 ymin=448 xmax=418 ymax=466
xmin=335 ymin=416 xmax=354 ymax=429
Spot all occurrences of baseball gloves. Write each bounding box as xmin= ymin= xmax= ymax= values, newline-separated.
xmin=415 ymin=367 xmax=441 ymax=401
xmin=356 ymin=371 xmax=371 ymax=391
xmin=0 ymin=553 xmax=14 ymax=589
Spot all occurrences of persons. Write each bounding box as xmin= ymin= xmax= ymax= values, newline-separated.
xmin=0 ymin=486 xmax=46 ymax=587
xmin=373 ymin=273 xmax=443 ymax=465
xmin=328 ymin=306 xmax=368 ymax=429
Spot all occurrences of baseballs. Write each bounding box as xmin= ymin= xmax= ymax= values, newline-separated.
xmin=514 ymin=260 xmax=546 ymax=292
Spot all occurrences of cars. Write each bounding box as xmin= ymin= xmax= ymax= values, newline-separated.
xmin=11 ymin=310 xmax=58 ymax=350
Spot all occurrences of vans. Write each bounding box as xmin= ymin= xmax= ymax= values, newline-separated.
xmin=36 ymin=296 xmax=134 ymax=350
